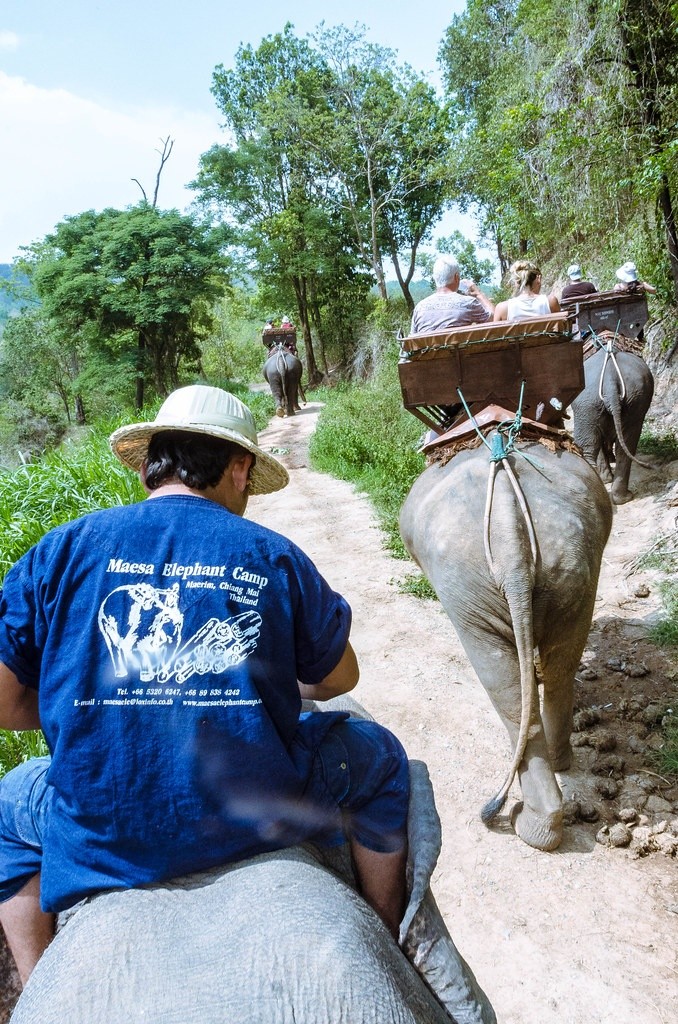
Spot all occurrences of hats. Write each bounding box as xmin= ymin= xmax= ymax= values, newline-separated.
xmin=268 ymin=319 xmax=274 ymax=323
xmin=616 ymin=262 xmax=639 ymax=282
xmin=282 ymin=316 xmax=289 ymax=323
xmin=567 ymin=265 xmax=581 ymax=281
xmin=109 ymin=383 xmax=290 ymax=496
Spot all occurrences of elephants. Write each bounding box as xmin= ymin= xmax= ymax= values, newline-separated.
xmin=263 ymin=344 xmax=308 ymax=418
xmin=2 ymin=682 xmax=501 ymax=1024
xmin=398 ymin=405 xmax=612 ymax=851
xmin=569 ymin=328 xmax=654 ymax=504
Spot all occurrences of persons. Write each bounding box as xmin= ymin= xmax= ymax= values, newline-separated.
xmin=0 ymin=385 xmax=411 ymax=986
xmin=562 ymin=265 xmax=596 ymax=299
xmin=281 ymin=316 xmax=292 ymax=328
xmin=411 ymin=255 xmax=494 ymax=334
xmin=493 ymin=261 xmax=560 ymax=321
xmin=614 ymin=262 xmax=656 ymax=293
